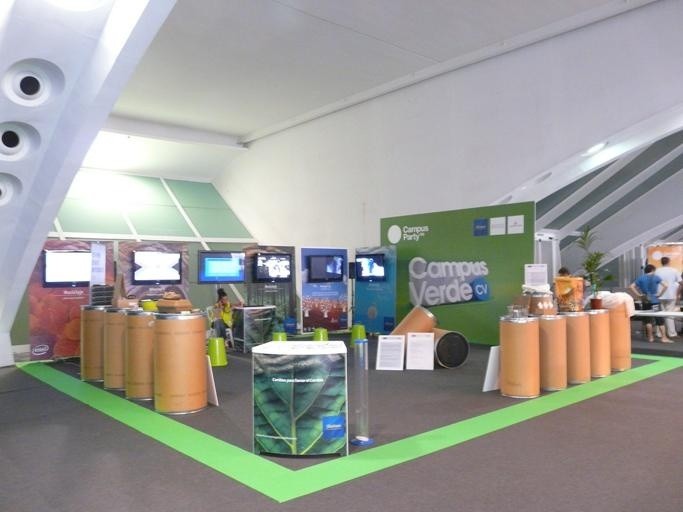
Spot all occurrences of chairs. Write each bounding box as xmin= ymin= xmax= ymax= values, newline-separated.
xmin=629 ymin=300 xmax=645 ymax=340
xmin=206 ymin=306 xmax=235 ymax=349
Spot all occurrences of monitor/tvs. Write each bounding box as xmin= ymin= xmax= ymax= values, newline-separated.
xmin=253 ymin=253 xmax=292 ymax=283
xmin=132 ymin=250 xmax=182 ymax=285
xmin=355 ymin=254 xmax=385 ymax=281
xmin=308 ymin=255 xmax=343 ymax=282
xmin=198 ymin=251 xmax=246 ymax=284
xmin=43 ymin=250 xmax=92 ymax=287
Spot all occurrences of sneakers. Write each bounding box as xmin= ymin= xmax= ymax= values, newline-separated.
xmin=661 ymin=339 xmax=674 ymax=345
xmin=649 ymin=339 xmax=656 ymax=343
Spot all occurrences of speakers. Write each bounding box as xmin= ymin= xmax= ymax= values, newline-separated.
xmin=349 ymin=263 xmax=355 ymax=278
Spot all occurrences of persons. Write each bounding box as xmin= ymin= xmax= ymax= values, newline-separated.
xmin=274 ymin=255 xmax=291 ymax=279
xmin=303 ymin=294 xmax=347 ymax=334
xmin=207 ymin=288 xmax=243 ymax=348
xmin=557 ymin=266 xmax=572 ymax=278
xmin=628 ymin=264 xmax=675 ymax=344
xmin=674 ymin=273 xmax=683 ymax=337
xmin=653 ymin=257 xmax=682 ymax=340
xmin=261 ymin=255 xmax=279 ymax=278
